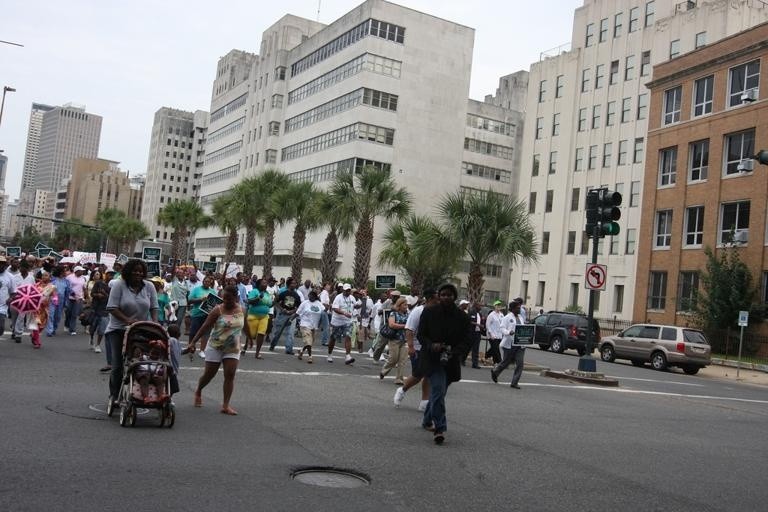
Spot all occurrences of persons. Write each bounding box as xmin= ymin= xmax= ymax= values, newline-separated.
xmin=379 ymin=297 xmax=411 ymax=386
xmin=392 ymin=288 xmax=440 ymax=411
xmin=490 ymin=300 xmax=527 ymax=391
xmin=188 ymin=282 xmax=255 ymax=416
xmin=103 ymin=260 xmax=161 ymax=409
xmin=164 ymin=323 xmax=195 ymax=419
xmin=0 ymin=244 xmax=530 ymax=374
xmin=135 ymin=338 xmax=170 ymax=406
xmin=415 ymin=283 xmax=476 ymax=445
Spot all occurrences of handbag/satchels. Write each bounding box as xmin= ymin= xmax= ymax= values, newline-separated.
xmin=440 ymin=344 xmax=462 ymax=382
xmin=79 ymin=308 xmax=95 ymax=326
xmin=380 ymin=324 xmax=398 ymax=339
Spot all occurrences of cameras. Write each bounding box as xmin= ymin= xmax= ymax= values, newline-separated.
xmin=440 ymin=343 xmax=453 ymax=365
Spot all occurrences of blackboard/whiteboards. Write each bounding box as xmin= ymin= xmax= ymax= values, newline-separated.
xmin=383 ymin=308 xmax=391 ymax=325
xmin=141 ymin=247 xmax=162 ymax=278
xmin=512 ymin=324 xmax=536 ymax=347
xmin=118 ymin=254 xmax=129 ymax=262
xmin=6 ymin=247 xmax=21 ymax=257
xmin=169 ymin=258 xmax=218 ymax=273
xmin=29 ymin=241 xmax=64 ymax=262
xmin=375 ymin=275 xmax=397 ymax=290
xmin=198 ymin=292 xmax=224 ymax=315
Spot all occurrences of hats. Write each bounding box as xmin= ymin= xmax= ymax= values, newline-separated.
xmin=338 ymin=282 xmax=343 ymax=287
xmin=391 ymin=290 xmax=401 ymax=296
xmin=148 ymin=276 xmax=161 ymax=286
xmin=269 ymin=277 xmax=278 ymax=283
xmin=494 ymin=301 xmax=502 ymax=307
xmin=343 ymin=284 xmax=351 ymax=291
xmin=74 ymin=266 xmax=85 ymax=273
xmin=460 ymin=300 xmax=470 ymax=305
xmin=105 ymin=269 xmax=117 ymax=274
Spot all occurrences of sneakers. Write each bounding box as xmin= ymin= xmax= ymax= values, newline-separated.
xmin=144 ymin=394 xmax=169 ymax=404
xmin=241 ymin=346 xmax=385 ymax=365
xmin=199 ymin=351 xmax=206 ymax=359
xmin=394 ymin=387 xmax=445 ymax=444
xmin=12 ymin=327 xmax=102 ymax=353
xmin=100 ymin=365 xmax=112 ymax=371
xmin=472 ymin=365 xmax=521 ymax=389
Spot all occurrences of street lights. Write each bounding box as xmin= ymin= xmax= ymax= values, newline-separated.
xmin=0 ymin=85 xmax=17 ymax=122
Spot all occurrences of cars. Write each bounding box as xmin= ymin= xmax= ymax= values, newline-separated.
xmin=477 ymin=305 xmax=504 ymax=335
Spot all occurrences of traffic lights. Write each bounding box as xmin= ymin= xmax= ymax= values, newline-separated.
xmin=599 ymin=189 xmax=621 ymax=237
xmin=584 ymin=191 xmax=597 ymax=238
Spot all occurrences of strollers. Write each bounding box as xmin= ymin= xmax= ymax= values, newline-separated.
xmin=103 ymin=320 xmax=177 ymax=429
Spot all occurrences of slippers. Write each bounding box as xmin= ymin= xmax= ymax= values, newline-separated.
xmin=195 ymin=392 xmax=202 ymax=407
xmin=221 ymin=406 xmax=238 ymax=415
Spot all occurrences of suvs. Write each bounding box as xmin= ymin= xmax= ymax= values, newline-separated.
xmin=522 ymin=310 xmax=601 ymax=357
xmin=596 ymin=323 xmax=712 ymax=375
